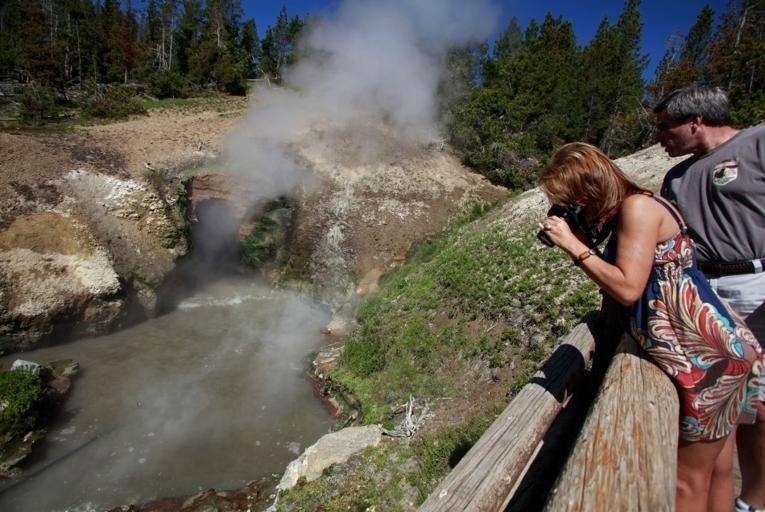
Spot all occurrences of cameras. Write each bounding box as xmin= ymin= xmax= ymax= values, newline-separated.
xmin=537 ymin=200 xmax=585 ymax=248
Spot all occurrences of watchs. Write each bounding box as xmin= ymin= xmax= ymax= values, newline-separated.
xmin=574 ymin=247 xmax=597 ymax=267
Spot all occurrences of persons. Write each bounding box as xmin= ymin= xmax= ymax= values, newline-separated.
xmin=535 ymin=141 xmax=764 ymax=511
xmin=649 ymin=82 xmax=765 ymax=511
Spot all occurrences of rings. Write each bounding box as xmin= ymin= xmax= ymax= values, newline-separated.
xmin=544 ymin=223 xmax=552 ymax=230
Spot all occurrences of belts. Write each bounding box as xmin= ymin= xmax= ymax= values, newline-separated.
xmin=706 ymin=260 xmax=765 ymax=280
